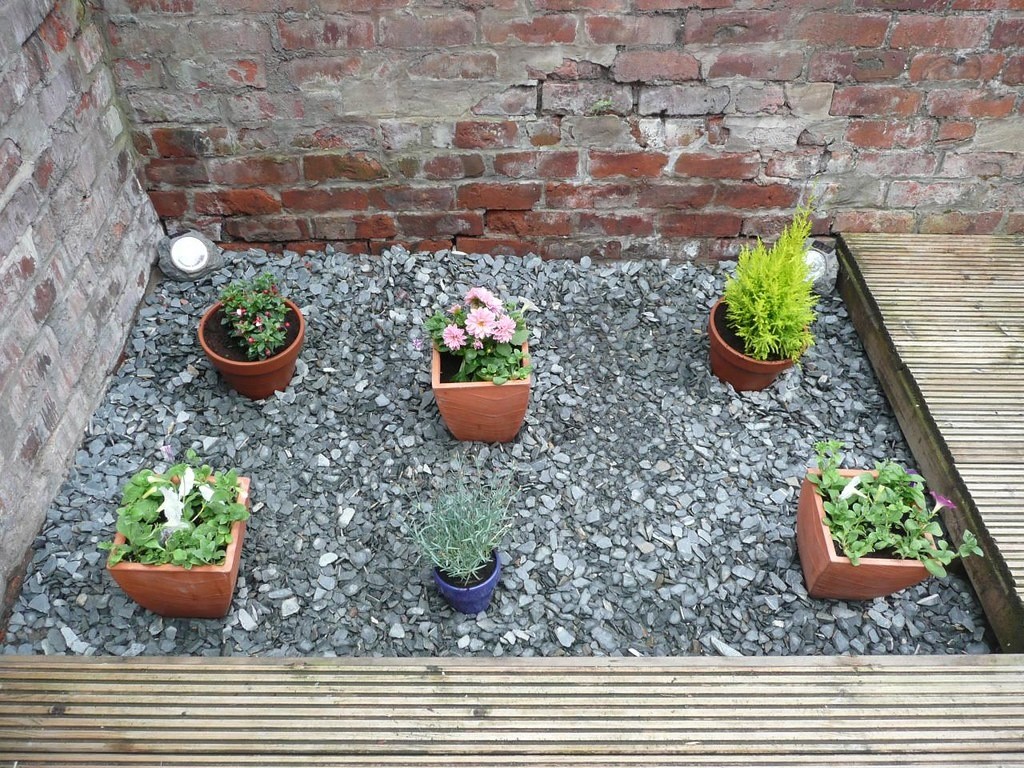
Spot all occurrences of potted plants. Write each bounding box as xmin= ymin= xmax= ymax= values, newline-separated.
xmin=394 ymin=444 xmax=522 ymax=614
xmin=709 ymin=177 xmax=819 ymax=392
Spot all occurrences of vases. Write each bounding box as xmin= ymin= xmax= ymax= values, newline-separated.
xmin=797 ymin=468 xmax=942 ymax=600
xmin=432 ymin=340 xmax=531 ymax=443
xmin=198 ymin=299 xmax=304 ymax=400
xmin=107 ymin=476 xmax=251 ymax=619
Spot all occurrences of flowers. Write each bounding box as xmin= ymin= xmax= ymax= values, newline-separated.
xmin=96 ymin=445 xmax=251 ymax=570
xmin=412 ymin=287 xmax=541 ymax=385
xmin=218 ymin=271 xmax=292 ymax=362
xmin=805 ymin=438 xmax=984 ymax=578
